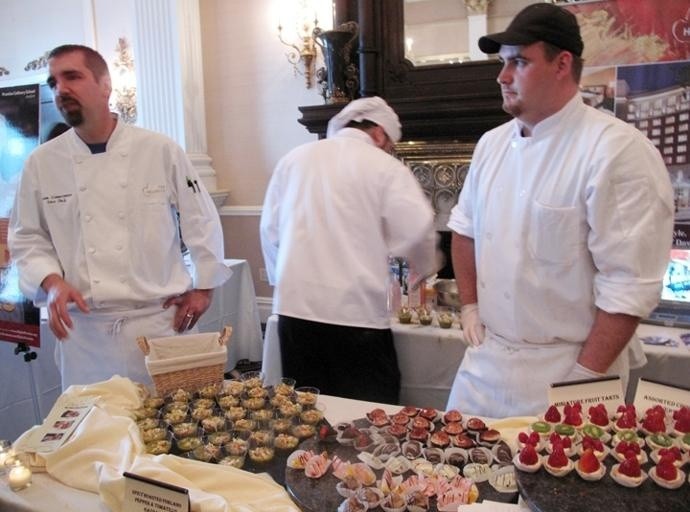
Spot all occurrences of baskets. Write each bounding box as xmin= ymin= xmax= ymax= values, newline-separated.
xmin=136 ymin=327 xmax=232 ymax=395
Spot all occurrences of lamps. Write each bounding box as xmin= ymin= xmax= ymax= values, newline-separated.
xmin=273 ymin=7 xmax=318 ymax=91
xmin=107 ymin=34 xmax=135 ymax=124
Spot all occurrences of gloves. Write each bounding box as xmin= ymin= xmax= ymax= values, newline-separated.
xmin=566 ymin=363 xmax=604 ymax=381
xmin=460 ymin=302 xmax=487 ymax=347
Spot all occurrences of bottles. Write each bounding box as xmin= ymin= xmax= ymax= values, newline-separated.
xmin=670 ymin=169 xmax=690 ymax=221
xmin=390 ymin=270 xmax=425 ymax=312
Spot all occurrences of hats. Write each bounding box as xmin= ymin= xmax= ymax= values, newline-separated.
xmin=478 ymin=4 xmax=579 ymax=54
xmin=327 ymin=96 xmax=403 ymax=145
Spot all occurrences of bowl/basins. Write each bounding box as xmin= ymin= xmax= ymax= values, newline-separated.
xmin=432 ymin=278 xmax=464 ymax=304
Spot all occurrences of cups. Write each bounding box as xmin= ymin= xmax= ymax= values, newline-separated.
xmin=0 ymin=439 xmax=34 ymax=491
xmin=400 ymin=302 xmax=466 ymax=330
xmin=134 ymin=372 xmax=327 ymax=474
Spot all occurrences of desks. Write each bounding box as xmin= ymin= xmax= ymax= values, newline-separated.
xmin=0 ymin=258 xmax=690 ymax=512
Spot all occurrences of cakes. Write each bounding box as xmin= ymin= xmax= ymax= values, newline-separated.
xmin=398 ymin=305 xmax=455 ymax=328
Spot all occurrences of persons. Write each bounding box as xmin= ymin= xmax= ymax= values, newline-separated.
xmin=259 ymin=96 xmax=442 ymax=412
xmin=440 ymin=2 xmax=675 ymax=424
xmin=6 ymin=47 xmax=234 ymax=400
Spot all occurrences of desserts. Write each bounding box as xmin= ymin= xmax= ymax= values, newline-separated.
xmin=136 ymin=380 xmax=326 ymax=468
xmin=512 ymin=401 xmax=689 ymax=489
xmin=286 ymin=405 xmax=520 ymax=510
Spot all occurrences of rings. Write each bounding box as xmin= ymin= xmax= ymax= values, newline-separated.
xmin=186 ymin=312 xmax=193 ymax=319
